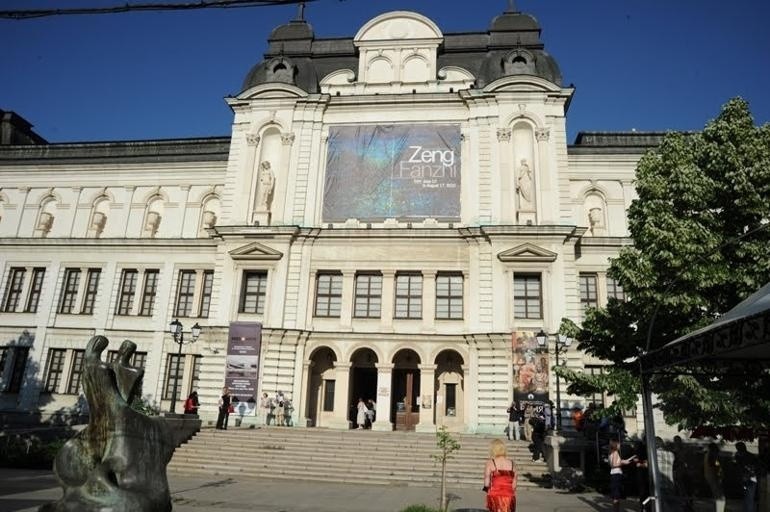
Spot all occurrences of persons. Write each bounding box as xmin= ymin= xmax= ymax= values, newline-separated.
xmin=216 ymin=385 xmax=232 ymax=430
xmin=483 ymin=438 xmax=519 ymax=512
xmin=516 ymin=158 xmax=534 ymax=210
xmin=273 ymin=390 xmax=285 ymax=426
xmin=515 ymin=335 xmax=548 ymax=396
xmin=506 ymin=400 xmax=521 ymax=440
xmin=190 ymin=390 xmax=200 ymax=415
xmin=528 ymin=413 xmax=548 ymax=463
xmin=702 ymin=442 xmax=729 ymax=511
xmin=259 ymin=392 xmax=271 ymax=424
xmin=605 ymin=433 xmax=695 ymax=511
xmin=523 ymin=402 xmax=534 ymax=441
xmin=572 ymin=400 xmax=627 ymax=433
xmin=733 ymin=441 xmax=761 ymax=511
xmin=255 ymin=161 xmax=275 ymax=211
xmin=184 ymin=392 xmax=200 ymax=415
xmin=352 ymin=397 xmax=377 ymax=430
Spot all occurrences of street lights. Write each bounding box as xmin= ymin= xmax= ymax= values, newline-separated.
xmin=532 ymin=325 xmax=572 ymax=434
xmin=166 ymin=318 xmax=203 ymax=414
